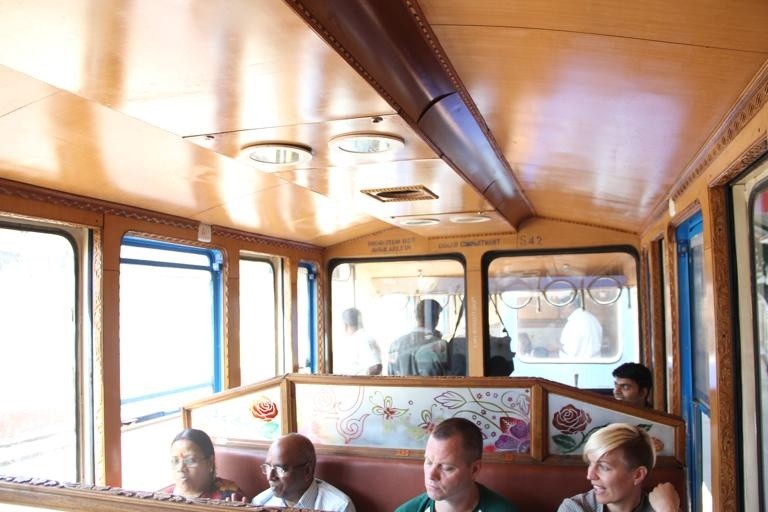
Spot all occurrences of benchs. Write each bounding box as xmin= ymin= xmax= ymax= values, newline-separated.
xmin=1 ymin=474 xmax=310 ymax=511
xmin=210 ymin=440 xmax=690 ymax=511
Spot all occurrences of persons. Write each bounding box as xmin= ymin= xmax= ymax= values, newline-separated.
xmin=612 ymin=363 xmax=653 ymax=408
xmin=518 ymin=294 xmax=603 ymax=360
xmin=224 ymin=434 xmax=357 ymax=512
xmin=342 ymin=308 xmax=382 ymax=375
xmin=389 ymin=418 xmax=519 ymax=512
xmin=154 ymin=429 xmax=246 ymax=502
xmin=558 ymin=422 xmax=681 ymax=512
xmin=388 ymin=299 xmax=449 ymax=377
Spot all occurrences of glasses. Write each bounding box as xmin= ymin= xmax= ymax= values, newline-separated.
xmin=170 ymin=456 xmax=209 ymax=466
xmin=260 ymin=463 xmax=308 ymax=477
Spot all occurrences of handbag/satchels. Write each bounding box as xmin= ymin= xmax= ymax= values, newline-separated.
xmin=448 ymin=336 xmax=515 ymax=376
